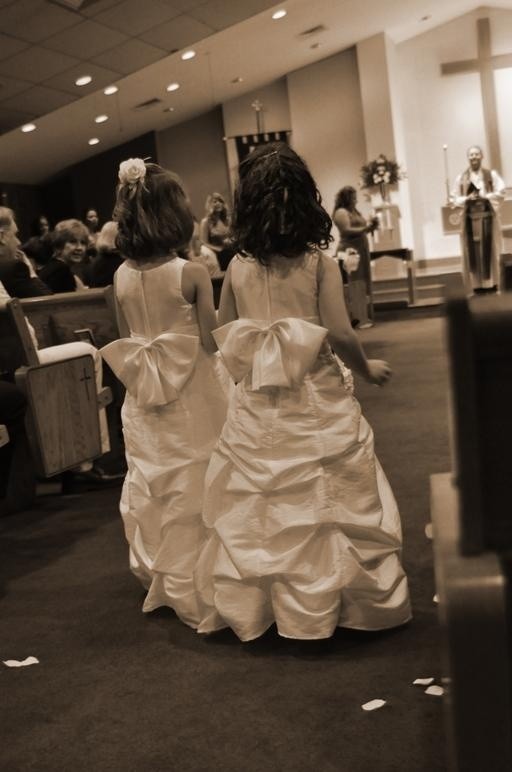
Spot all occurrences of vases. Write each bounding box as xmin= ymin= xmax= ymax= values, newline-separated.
xmin=378 ymin=182 xmax=391 ymax=205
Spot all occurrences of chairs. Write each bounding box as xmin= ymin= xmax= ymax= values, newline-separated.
xmin=0 ymin=260 xmax=117 ymax=480
xmin=425 ymin=282 xmax=511 ymax=771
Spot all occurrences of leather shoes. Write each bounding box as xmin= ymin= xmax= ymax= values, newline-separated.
xmin=72 ymin=463 xmax=128 ymax=493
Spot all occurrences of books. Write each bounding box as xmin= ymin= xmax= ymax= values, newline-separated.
xmin=465 ymin=182 xmax=480 ymax=204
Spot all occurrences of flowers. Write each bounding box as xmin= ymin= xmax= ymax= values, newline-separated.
xmin=358 ymin=154 xmax=402 ymax=187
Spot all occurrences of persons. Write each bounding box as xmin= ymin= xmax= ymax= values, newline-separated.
xmin=188 ymin=190 xmax=234 ymax=274
xmin=0 ymin=205 xmax=126 ymax=303
xmin=93 ymin=155 xmax=234 ymax=631
xmin=189 ymin=137 xmax=420 ymax=645
xmin=446 ymin=144 xmax=509 ymax=298
xmin=332 ymin=185 xmax=381 ymax=329
